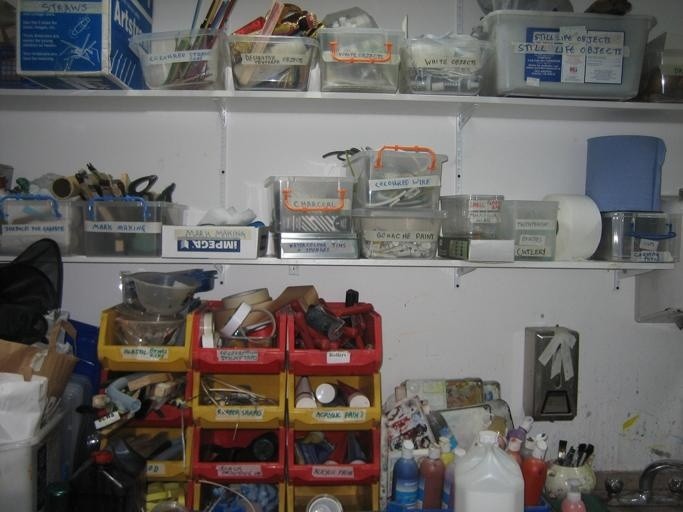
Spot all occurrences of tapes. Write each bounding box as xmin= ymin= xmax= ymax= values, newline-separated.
xmin=220 ymin=288 xmax=269 ymax=337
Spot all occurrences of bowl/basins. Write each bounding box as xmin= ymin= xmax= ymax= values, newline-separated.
xmin=117 ymin=316 xmax=182 ymax=346
xmin=130 ymin=271 xmax=199 ymax=315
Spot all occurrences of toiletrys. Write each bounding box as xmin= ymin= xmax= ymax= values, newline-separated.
xmin=314 ymin=381 xmax=340 ymax=406
xmin=295 ymin=376 xmax=318 ymax=408
xmin=346 ymin=432 xmax=371 ymax=464
xmin=324 ymin=441 xmax=346 ymax=466
xmin=336 ymin=378 xmax=371 ymax=407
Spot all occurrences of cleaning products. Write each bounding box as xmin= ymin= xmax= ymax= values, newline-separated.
xmin=383 ymin=414 xmax=589 ymax=512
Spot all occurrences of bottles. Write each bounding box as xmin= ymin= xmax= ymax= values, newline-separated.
xmin=500 ymin=416 xmax=548 ymax=506
xmin=391 ymin=436 xmax=465 ymax=512
xmin=561 ymin=492 xmax=585 ymax=511
xmin=294 ymin=372 xmax=368 ymax=408
xmin=45 ymin=447 xmax=136 ymax=509
xmin=543 ymin=462 xmax=595 ymax=497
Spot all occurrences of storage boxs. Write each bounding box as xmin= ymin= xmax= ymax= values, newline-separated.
xmin=595 ymin=211 xmax=670 ymax=265
xmin=437 ymin=193 xmax=505 ymax=237
xmin=437 ymin=236 xmax=516 ymax=263
xmin=638 ymin=49 xmax=678 ymax=104
xmin=266 ymin=175 xmax=359 ymax=232
xmin=228 ymin=35 xmax=318 ymax=92
xmin=128 ymin=30 xmax=227 ymax=91
xmin=504 ymin=200 xmax=560 ymax=260
xmin=343 ymin=145 xmax=449 ymax=212
xmin=1 ymin=196 xmax=81 ymax=255
xmin=318 ymin=31 xmax=404 ymax=95
xmin=74 ymin=198 xmax=186 ymax=257
xmin=12 ymin=0 xmax=154 ymax=89
xmin=278 ymin=229 xmax=360 ymax=259
xmin=398 ymin=37 xmax=491 ymax=96
xmin=0 ymin=400 xmax=73 ymax=510
xmin=353 ymin=209 xmax=448 ymax=258
xmin=474 ymin=11 xmax=656 ymax=100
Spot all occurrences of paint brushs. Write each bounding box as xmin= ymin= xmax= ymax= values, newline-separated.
xmin=558 ymin=440 xmax=594 ymax=467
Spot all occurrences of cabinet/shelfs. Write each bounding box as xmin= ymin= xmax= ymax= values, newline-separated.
xmin=94 ymin=303 xmax=386 ymax=511
xmin=0 ymin=85 xmax=683 ymax=293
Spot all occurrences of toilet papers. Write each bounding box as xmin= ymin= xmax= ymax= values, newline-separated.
xmin=545 ymin=193 xmax=602 ymax=260
